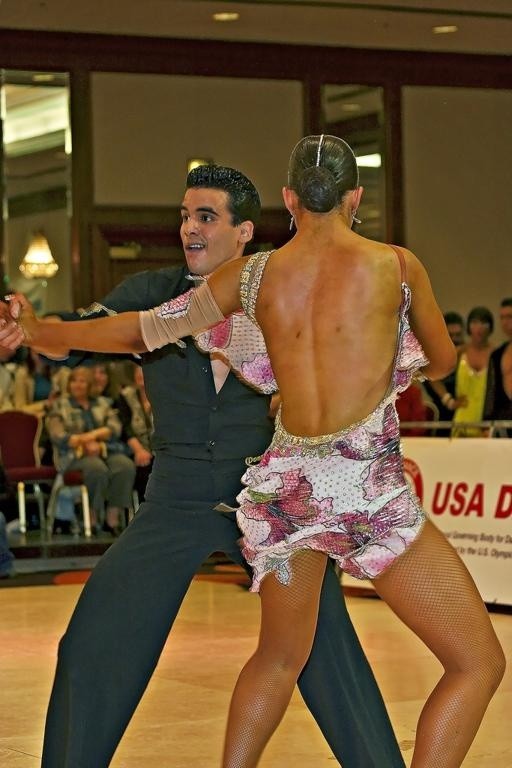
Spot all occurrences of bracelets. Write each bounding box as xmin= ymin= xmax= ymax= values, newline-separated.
xmin=445 ymin=395 xmax=456 ymax=411
xmin=442 ymin=392 xmax=450 ymax=406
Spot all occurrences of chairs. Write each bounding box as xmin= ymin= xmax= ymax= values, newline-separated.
xmin=47 ymin=442 xmax=140 ymax=538
xmin=0 ymin=411 xmax=52 ymax=534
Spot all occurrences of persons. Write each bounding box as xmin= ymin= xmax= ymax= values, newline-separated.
xmin=1 ymin=302 xmax=155 ymax=536
xmin=479 ymin=297 xmax=510 ymax=437
xmin=1 ymin=160 xmax=406 ymax=767
xmin=0 ymin=126 xmax=508 ymax=767
xmin=427 ymin=307 xmax=498 ymax=438
xmin=426 ymin=311 xmax=466 ymax=436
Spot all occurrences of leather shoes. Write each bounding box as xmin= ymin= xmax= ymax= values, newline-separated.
xmin=101 ymin=520 xmax=122 ymax=537
xmin=83 ymin=525 xmax=100 ymax=535
